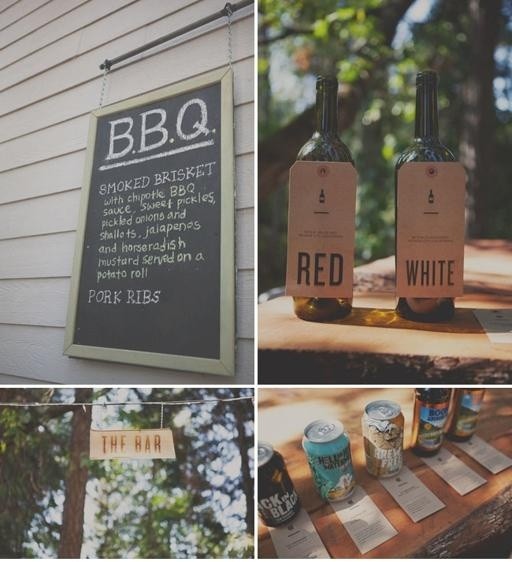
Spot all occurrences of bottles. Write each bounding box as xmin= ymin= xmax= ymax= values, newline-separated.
xmin=393 ymin=70 xmax=460 ymax=324
xmin=289 ymin=74 xmax=355 ymax=323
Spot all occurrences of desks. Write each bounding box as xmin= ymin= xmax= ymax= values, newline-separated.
xmin=258 ymin=385 xmax=512 ymax=559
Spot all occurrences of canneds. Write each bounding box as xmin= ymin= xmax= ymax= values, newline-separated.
xmin=301 ymin=419 xmax=357 ymax=502
xmin=257 ymin=441 xmax=300 ymax=528
xmin=361 ymin=400 xmax=404 ymax=478
